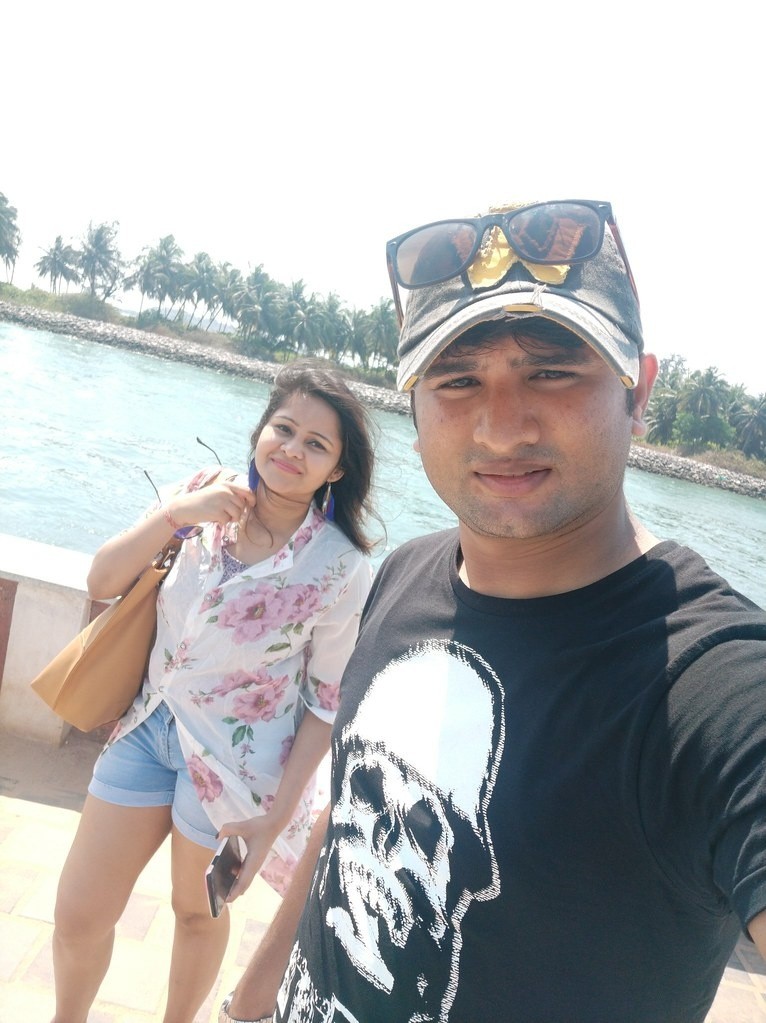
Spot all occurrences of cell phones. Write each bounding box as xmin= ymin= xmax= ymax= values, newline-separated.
xmin=204 ymin=835 xmax=242 ymax=919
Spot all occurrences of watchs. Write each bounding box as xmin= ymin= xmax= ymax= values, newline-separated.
xmin=217 ymin=988 xmax=280 ymax=1023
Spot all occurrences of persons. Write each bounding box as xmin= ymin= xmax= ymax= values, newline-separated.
xmin=52 ymin=368 xmax=376 ymax=1023
xmin=213 ymin=200 xmax=764 ymax=1022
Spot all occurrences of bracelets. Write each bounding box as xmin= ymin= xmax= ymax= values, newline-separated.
xmin=162 ymin=502 xmax=187 ymax=530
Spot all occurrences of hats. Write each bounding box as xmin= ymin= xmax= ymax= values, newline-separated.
xmin=396 ymin=200 xmax=645 ymax=392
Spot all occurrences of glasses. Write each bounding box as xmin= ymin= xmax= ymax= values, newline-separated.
xmin=143 ymin=436 xmax=228 ymax=540
xmin=385 ymin=200 xmax=640 ymax=334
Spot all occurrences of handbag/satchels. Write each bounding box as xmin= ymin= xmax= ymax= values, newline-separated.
xmin=30 ymin=465 xmax=223 ymax=733
